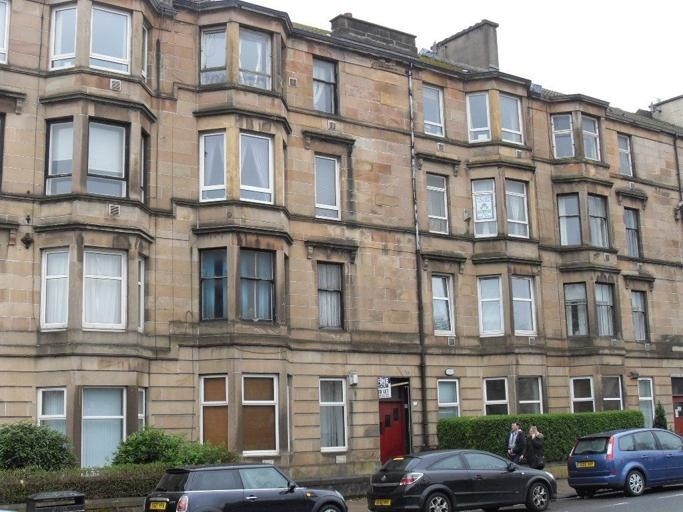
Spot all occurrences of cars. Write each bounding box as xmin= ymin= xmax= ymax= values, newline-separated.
xmin=367 ymin=451 xmax=557 ymax=512
xmin=144 ymin=465 xmax=348 ymax=512
xmin=568 ymin=428 xmax=683 ymax=496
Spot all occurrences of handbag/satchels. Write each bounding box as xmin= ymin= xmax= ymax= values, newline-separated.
xmin=536 ymin=456 xmax=545 ymax=468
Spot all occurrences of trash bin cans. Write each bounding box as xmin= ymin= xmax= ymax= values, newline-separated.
xmin=26 ymin=491 xmax=86 ymax=512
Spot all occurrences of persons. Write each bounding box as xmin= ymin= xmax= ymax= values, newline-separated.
xmin=518 ymin=424 xmax=545 ymax=469
xmin=504 ymin=421 xmax=524 ymax=464
